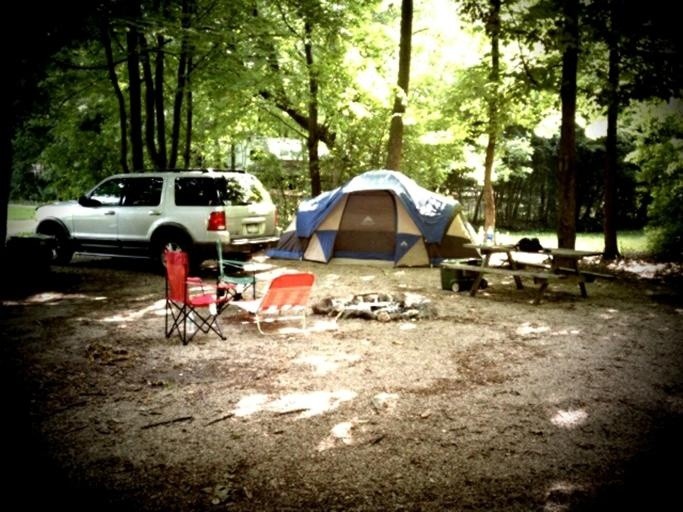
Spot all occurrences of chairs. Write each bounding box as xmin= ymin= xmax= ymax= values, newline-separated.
xmin=214 ymin=240 xmax=256 ymax=301
xmin=229 ymin=273 xmax=314 ymax=336
xmin=161 ymin=250 xmax=227 ymax=343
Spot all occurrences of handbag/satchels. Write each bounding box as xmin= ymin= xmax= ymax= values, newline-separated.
xmin=513 ymin=238 xmax=550 ymax=253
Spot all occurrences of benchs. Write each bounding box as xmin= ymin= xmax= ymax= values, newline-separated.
xmin=501 ymin=258 xmax=614 ymax=297
xmin=445 ymin=263 xmax=566 ymax=305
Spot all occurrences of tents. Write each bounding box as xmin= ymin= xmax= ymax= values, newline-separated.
xmin=265 ymin=168 xmax=483 ymax=268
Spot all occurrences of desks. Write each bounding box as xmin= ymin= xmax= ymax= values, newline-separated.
xmin=462 ymin=242 xmax=604 ymax=305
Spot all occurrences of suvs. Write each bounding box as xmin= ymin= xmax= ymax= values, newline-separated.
xmin=29 ymin=166 xmax=284 ymax=277
